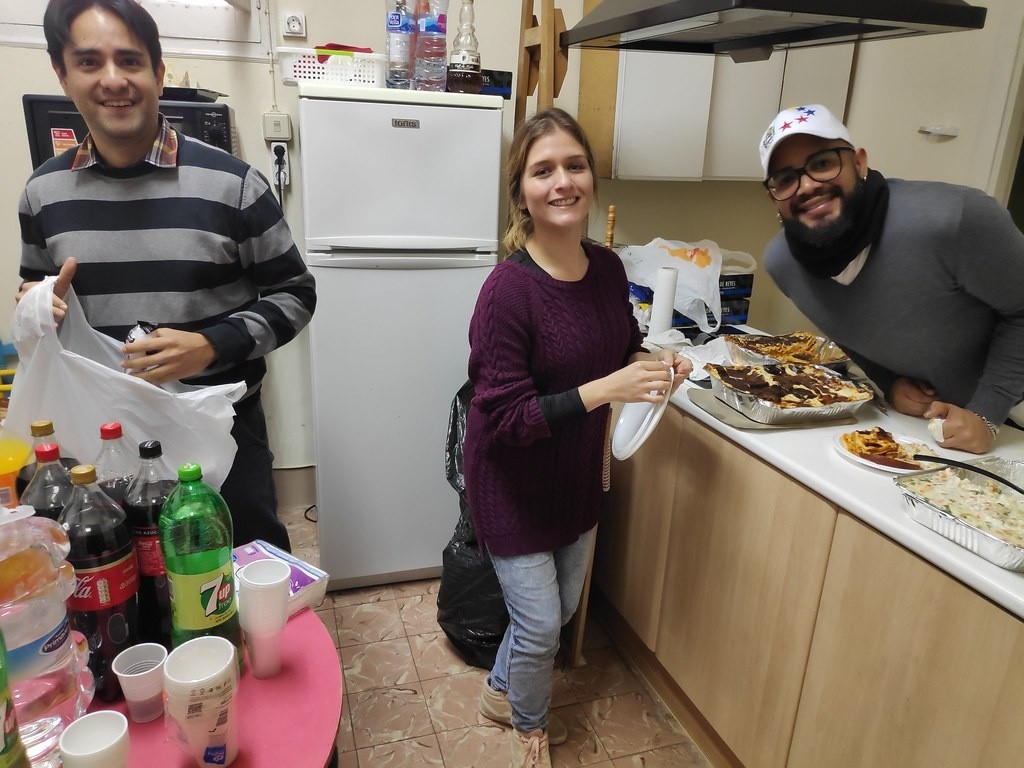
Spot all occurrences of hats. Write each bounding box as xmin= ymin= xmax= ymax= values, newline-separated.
xmin=759 ymin=104 xmax=855 ymax=178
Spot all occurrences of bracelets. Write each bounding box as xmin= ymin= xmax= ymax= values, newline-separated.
xmin=974 ymin=413 xmax=999 ymax=440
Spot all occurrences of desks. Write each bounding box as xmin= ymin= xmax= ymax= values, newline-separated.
xmin=60 ymin=606 xmax=343 ymax=767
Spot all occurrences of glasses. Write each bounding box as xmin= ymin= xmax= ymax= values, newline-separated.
xmin=762 ymin=146 xmax=856 ymax=202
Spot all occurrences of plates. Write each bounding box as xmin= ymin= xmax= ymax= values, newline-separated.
xmin=833 ymin=430 xmax=948 ymax=475
xmin=611 ymin=366 xmax=676 ymax=462
xmin=160 ymin=86 xmax=230 ymax=103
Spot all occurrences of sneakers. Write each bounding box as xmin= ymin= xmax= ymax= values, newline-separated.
xmin=510 ymin=726 xmax=552 ymax=768
xmin=479 ymin=675 xmax=566 ymax=744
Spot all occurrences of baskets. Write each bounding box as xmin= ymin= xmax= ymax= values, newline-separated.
xmin=276 ymin=46 xmax=388 ymax=88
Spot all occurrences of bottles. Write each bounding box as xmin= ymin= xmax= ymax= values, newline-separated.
xmin=92 ymin=421 xmax=140 ymax=504
xmin=55 ymin=463 xmax=146 ymax=699
xmin=0 ymin=627 xmax=32 ymax=768
xmin=160 ymin=462 xmax=244 ymax=672
xmin=447 ymin=0 xmax=483 ymax=93
xmin=385 ymin=0 xmax=416 ymax=90
xmin=414 ymin=0 xmax=449 ymax=93
xmin=17 ymin=420 xmax=82 ymax=498
xmin=0 ymin=428 xmax=30 ymax=509
xmin=1 ymin=506 xmax=93 ymax=768
xmin=19 ymin=442 xmax=76 ymax=524
xmin=409 ymin=0 xmax=446 ymax=92
xmin=121 ymin=440 xmax=180 ymax=649
xmin=124 ymin=318 xmax=158 ymax=388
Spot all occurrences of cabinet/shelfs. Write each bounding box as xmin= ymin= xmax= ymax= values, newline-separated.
xmin=574 ymin=0 xmax=1024 ymax=218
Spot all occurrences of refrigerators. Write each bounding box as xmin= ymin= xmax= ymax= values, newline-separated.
xmin=295 ymin=81 xmax=506 ymax=598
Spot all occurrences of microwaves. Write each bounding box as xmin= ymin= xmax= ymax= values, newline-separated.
xmin=22 ymin=93 xmax=237 ymax=175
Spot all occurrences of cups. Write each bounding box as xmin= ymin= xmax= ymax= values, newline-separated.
xmin=59 ymin=559 xmax=290 ymax=768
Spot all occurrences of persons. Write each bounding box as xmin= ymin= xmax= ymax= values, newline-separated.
xmin=15 ymin=0 xmax=318 ymax=555
xmin=758 ymin=104 xmax=1024 ymax=454
xmin=461 ymin=107 xmax=694 ymax=768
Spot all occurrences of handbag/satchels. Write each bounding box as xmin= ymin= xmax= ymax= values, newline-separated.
xmin=621 ymin=237 xmax=757 ymax=333
xmin=3 ymin=276 xmax=247 ymax=494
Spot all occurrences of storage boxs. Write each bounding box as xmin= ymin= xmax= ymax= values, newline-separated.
xmin=628 ymin=274 xmax=754 ymax=327
